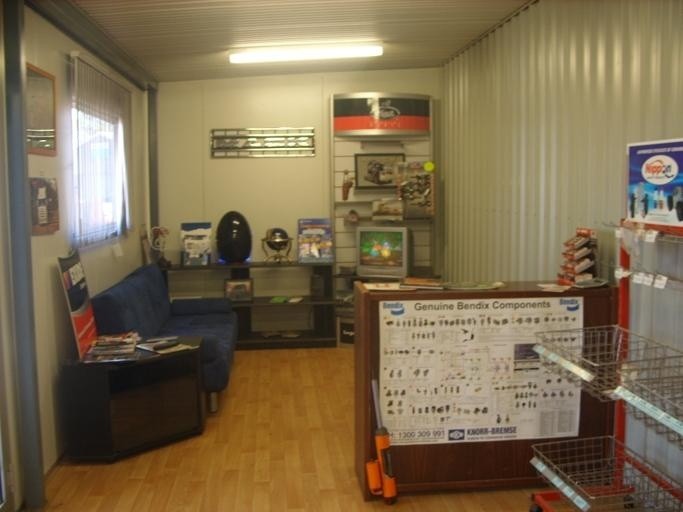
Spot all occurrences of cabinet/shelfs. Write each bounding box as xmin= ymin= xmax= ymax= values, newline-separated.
xmin=327 ymin=92 xmax=440 ymax=350
xmin=527 ymin=321 xmax=681 ymax=511
xmin=154 ymin=261 xmax=335 ymax=348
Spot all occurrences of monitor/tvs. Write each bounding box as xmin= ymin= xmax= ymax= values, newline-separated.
xmin=355 ymin=227 xmax=414 ymax=278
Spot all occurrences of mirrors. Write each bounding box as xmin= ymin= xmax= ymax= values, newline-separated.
xmin=25 ymin=61 xmax=57 ymax=158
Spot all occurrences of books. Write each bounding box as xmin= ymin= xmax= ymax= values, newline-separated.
xmin=281 ymin=332 xmax=300 ymax=337
xmin=289 ymin=297 xmax=303 ymax=303
xmin=270 ymin=296 xmax=287 ymax=303
xmin=399 ymin=276 xmax=444 ymax=290
xmin=264 ymin=331 xmax=280 ymax=337
xmin=87 ymin=333 xmax=192 ymax=354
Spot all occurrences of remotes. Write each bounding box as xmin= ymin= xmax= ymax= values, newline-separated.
xmin=153 ymin=340 xmax=179 ymax=350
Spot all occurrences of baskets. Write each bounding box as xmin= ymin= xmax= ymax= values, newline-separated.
xmin=532 ymin=436 xmax=683 ymax=512
xmin=620 ymin=365 xmax=683 ymax=450
xmin=533 ymin=325 xmax=683 ymax=401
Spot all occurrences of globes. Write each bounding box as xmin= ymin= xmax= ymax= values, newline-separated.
xmin=261 ymin=228 xmax=294 ymax=262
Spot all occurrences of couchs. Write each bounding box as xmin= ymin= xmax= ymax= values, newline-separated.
xmin=90 ymin=262 xmax=238 ymax=415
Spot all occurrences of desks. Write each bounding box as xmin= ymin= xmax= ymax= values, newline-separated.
xmin=67 ymin=336 xmax=206 ymax=459
xmin=353 ymin=278 xmax=617 ymax=504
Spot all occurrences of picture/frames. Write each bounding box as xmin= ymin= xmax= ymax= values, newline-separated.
xmin=225 ymin=277 xmax=253 ymax=304
xmin=354 ymin=152 xmax=406 ymax=189
